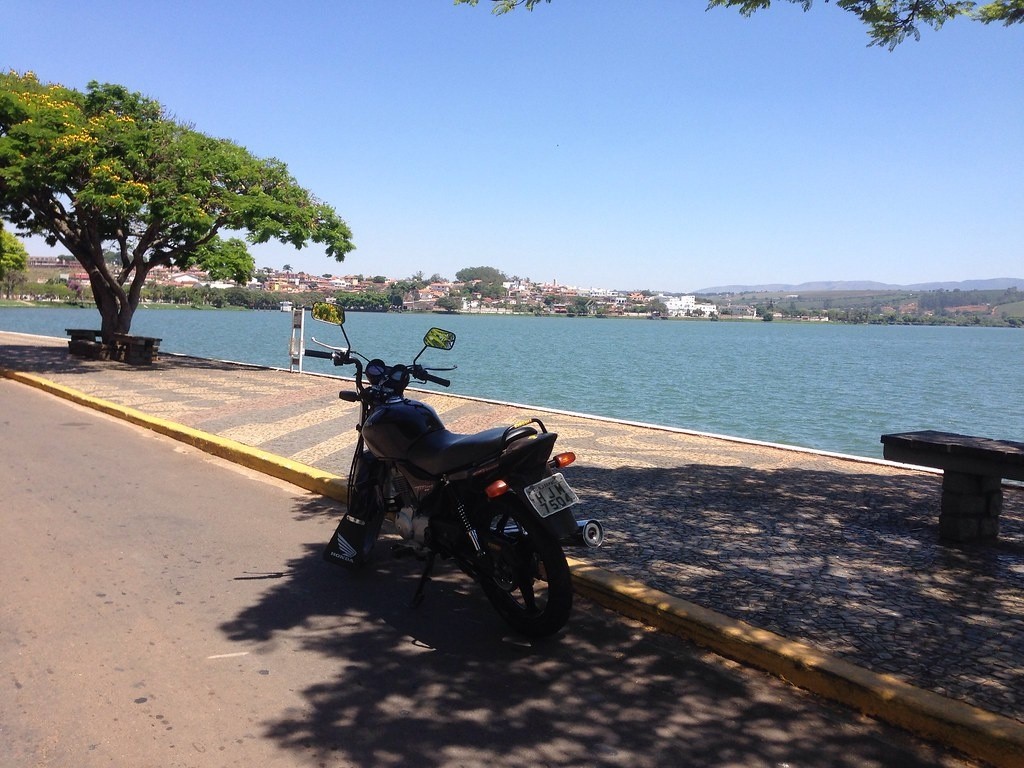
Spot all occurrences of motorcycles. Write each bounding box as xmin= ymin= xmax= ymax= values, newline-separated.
xmin=299 ymin=301 xmax=606 ymax=642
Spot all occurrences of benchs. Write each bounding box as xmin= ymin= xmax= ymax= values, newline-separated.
xmin=65 ymin=329 xmax=102 ymax=341
xmin=880 ymin=430 xmax=1024 ymax=547
xmin=116 ymin=332 xmax=162 ymax=364
